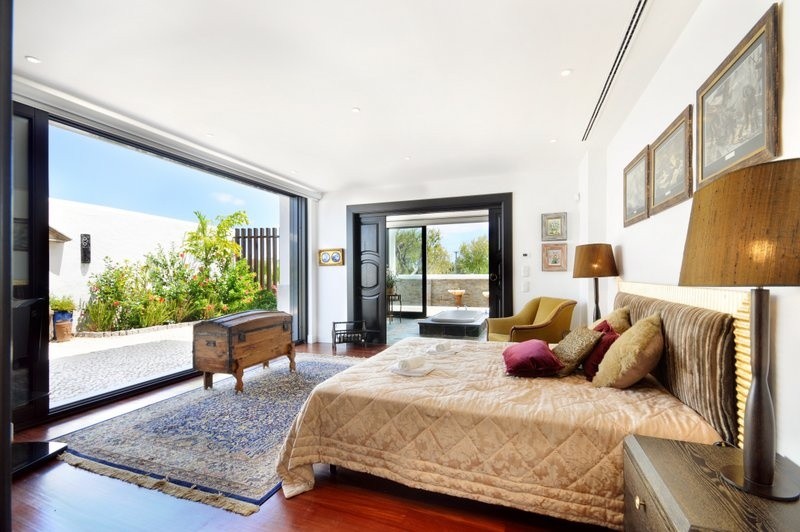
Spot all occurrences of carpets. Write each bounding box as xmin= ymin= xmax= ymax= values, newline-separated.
xmin=43 ymin=348 xmax=368 ymax=517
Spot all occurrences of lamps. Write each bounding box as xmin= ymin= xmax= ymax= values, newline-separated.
xmin=676 ymin=157 xmax=800 ymax=503
xmin=573 ymin=243 xmax=620 ymax=321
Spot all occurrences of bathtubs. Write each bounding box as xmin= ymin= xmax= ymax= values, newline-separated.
xmin=416 ymin=308 xmax=488 ymax=338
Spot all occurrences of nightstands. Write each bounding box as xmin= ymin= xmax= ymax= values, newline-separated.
xmin=623 ymin=433 xmax=800 ymax=532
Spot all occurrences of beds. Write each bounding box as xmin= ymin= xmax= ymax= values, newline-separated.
xmin=276 ymin=279 xmax=734 ymax=532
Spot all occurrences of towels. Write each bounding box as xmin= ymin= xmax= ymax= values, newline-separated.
xmin=391 ymin=361 xmax=437 ymax=376
xmin=397 ymin=356 xmax=425 ymax=371
xmin=435 ymin=340 xmax=451 ymax=351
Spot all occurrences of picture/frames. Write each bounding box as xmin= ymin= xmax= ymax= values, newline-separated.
xmin=541 ymin=244 xmax=568 ymax=272
xmin=541 ymin=213 xmax=568 ymax=240
xmin=696 ymin=2 xmax=782 ymax=190
xmin=623 ymin=145 xmax=649 ymax=227
xmin=649 ymin=104 xmax=693 ymax=216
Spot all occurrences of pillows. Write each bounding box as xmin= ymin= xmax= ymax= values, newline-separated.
xmin=502 ymin=308 xmax=667 ymax=389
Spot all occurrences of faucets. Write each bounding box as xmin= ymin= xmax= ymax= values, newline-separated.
xmin=463 ymin=303 xmax=468 ymax=310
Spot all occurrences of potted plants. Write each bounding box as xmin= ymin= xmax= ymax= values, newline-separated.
xmin=50 ymin=295 xmax=76 ymax=340
xmin=385 ymin=270 xmax=397 ymax=294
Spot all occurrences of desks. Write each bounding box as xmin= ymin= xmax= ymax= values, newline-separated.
xmin=386 ymin=295 xmax=402 ymax=325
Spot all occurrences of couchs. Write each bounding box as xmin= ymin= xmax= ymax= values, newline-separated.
xmin=487 ymin=295 xmax=577 ymax=343
